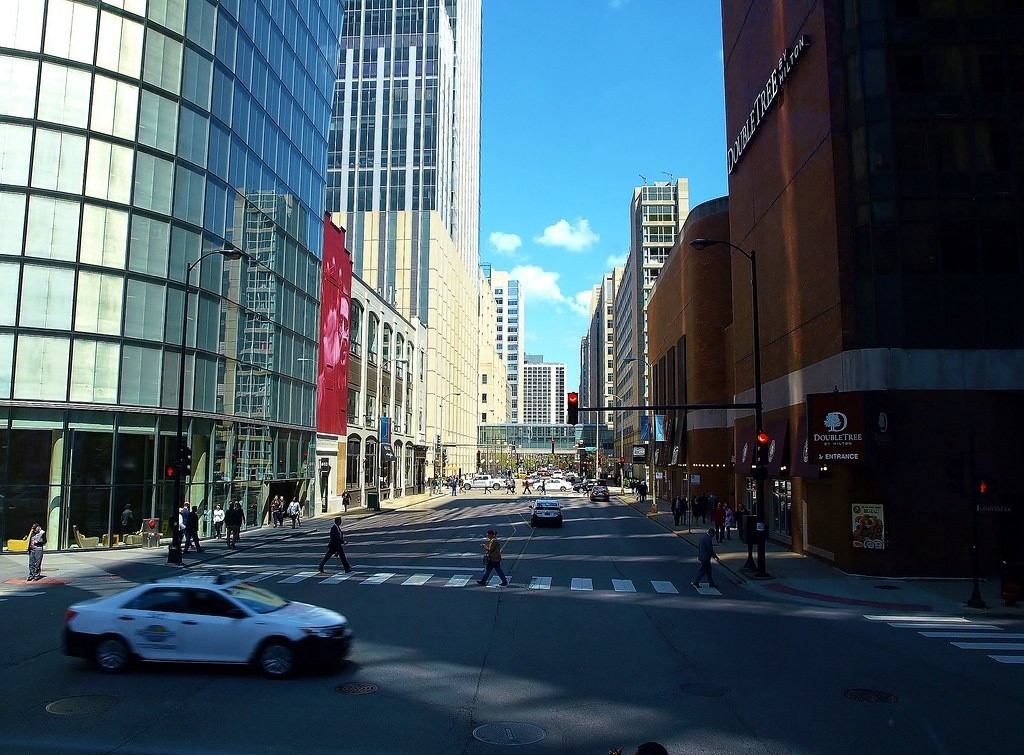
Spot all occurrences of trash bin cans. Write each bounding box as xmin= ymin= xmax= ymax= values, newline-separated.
xmin=367 ymin=492 xmax=380 ymax=511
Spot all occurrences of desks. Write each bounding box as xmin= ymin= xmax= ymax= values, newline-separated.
xmin=102 ymin=534 xmax=119 ymax=546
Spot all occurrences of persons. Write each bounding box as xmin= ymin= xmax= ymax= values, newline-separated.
xmin=27 ymin=523 xmax=47 ymax=581
xmin=179 ymin=500 xmax=245 ymax=554
xmin=317 ymin=295 xmax=350 ymax=435
xmin=609 ymin=742 xmax=670 ymax=755
xmin=476 ymin=530 xmax=507 ymax=586
xmin=202 ymin=495 xmax=257 ymax=535
xmin=693 ymin=529 xmax=721 ymax=589
xmin=671 ymin=492 xmax=750 ymax=543
xmin=342 ymin=489 xmax=351 ymax=512
xmin=431 ymin=472 xmax=648 ymax=502
xmin=270 ymin=495 xmax=301 ymax=529
xmin=317 ymin=517 xmax=352 ymax=574
xmin=120 ymin=504 xmax=136 ymax=534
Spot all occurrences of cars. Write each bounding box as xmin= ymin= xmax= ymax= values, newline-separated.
xmin=61 ymin=577 xmax=353 ymax=682
xmin=522 ymin=469 xmax=610 ymax=529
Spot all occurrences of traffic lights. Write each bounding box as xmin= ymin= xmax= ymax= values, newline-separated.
xmin=756 ymin=432 xmax=769 ymax=465
xmin=977 ymin=479 xmax=993 ymax=498
xmin=620 ymin=458 xmax=624 ymax=467
xmin=436 ymin=435 xmax=447 ymax=461
xmin=756 ymin=467 xmax=769 ymax=481
xmin=568 ymin=393 xmax=578 ymax=424
xmin=598 ymin=455 xmax=602 ymax=464
xmin=165 ymin=465 xmax=178 ymax=479
xmin=180 ymin=446 xmax=192 ymax=476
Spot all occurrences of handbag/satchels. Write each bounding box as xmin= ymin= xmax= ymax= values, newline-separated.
xmin=167 ymin=544 xmax=183 ymax=564
xmin=483 ymin=550 xmax=490 ymax=565
xmin=698 ymin=562 xmax=703 ymax=573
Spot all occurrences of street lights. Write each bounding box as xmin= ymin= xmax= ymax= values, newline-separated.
xmin=439 ymin=393 xmax=461 ymax=494
xmin=689 ymin=238 xmax=770 ymax=577
xmin=374 ymin=359 xmax=408 ymax=511
xmin=603 ymin=394 xmax=624 ymax=494
xmin=167 ymin=248 xmax=244 ymax=564
xmin=582 ymin=406 xmax=598 ymax=479
xmin=623 ymin=358 xmax=656 ymax=504
xmin=480 ymin=409 xmax=494 ymax=460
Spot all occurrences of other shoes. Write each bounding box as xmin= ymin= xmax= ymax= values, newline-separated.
xmin=693 ymin=582 xmax=702 ymax=589
xmin=499 ymin=582 xmax=508 ymax=585
xmin=477 ymin=580 xmax=486 ymax=585
xmin=318 ymin=567 xmax=326 ymax=573
xmin=345 ymin=570 xmax=353 ymax=573
xmin=709 ymin=583 xmax=718 ymax=587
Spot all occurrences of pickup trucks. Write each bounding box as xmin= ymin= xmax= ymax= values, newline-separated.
xmin=463 ymin=474 xmax=507 ymax=490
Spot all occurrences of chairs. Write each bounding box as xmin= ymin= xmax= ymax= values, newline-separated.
xmin=123 ymin=523 xmax=144 ymax=545
xmin=73 ymin=525 xmax=99 ymax=548
xmin=6 ymin=527 xmax=33 ymax=552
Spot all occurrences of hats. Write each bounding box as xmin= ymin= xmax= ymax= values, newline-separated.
xmin=32 ymin=523 xmax=39 ymax=529
